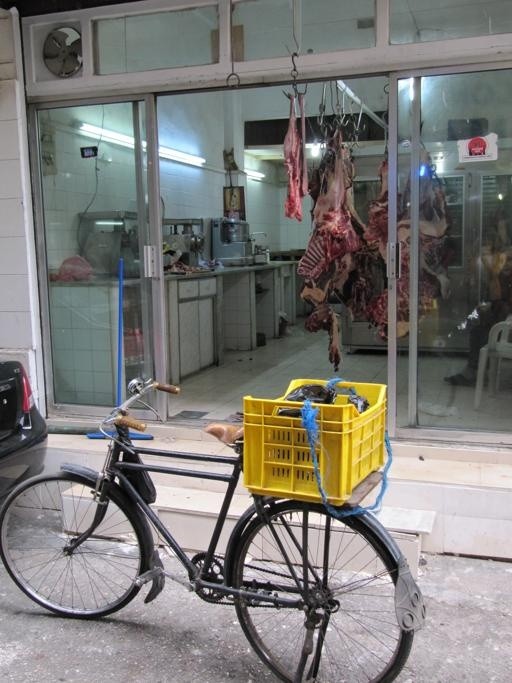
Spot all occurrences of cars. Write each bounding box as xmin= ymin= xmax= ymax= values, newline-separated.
xmin=0 ymin=358 xmax=48 ymax=502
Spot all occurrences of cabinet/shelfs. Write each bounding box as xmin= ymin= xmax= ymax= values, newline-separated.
xmin=340 ymin=173 xmax=469 ymax=355
xmin=48 ymin=260 xmax=312 ymax=408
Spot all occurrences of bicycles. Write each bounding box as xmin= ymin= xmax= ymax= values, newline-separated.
xmin=0 ymin=372 xmax=429 ymax=682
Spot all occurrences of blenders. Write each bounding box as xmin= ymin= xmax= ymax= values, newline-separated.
xmin=211 ymin=218 xmax=254 ymax=266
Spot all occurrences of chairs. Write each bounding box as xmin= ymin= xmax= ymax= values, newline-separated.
xmin=471 ymin=313 xmax=511 ymax=411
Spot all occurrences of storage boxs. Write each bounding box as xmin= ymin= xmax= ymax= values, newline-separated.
xmin=241 ymin=377 xmax=388 ymax=507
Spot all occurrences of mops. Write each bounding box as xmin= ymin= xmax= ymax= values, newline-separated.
xmin=85 ymin=257 xmax=155 ymax=442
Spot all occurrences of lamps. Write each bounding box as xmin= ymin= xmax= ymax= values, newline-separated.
xmin=77 ymin=119 xmax=206 ymax=167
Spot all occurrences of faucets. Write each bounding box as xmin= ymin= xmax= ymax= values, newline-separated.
xmin=249 ymin=230 xmax=269 ymax=241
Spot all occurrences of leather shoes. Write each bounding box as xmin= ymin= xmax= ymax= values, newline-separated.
xmin=444 ymin=372 xmax=476 ymax=386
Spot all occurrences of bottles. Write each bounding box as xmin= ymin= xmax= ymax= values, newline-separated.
xmin=256 ymin=280 xmax=264 ymax=293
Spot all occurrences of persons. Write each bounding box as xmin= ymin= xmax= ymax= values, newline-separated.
xmin=443 ymin=214 xmax=512 ymax=388
